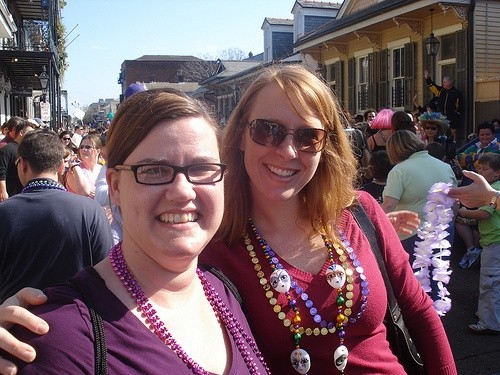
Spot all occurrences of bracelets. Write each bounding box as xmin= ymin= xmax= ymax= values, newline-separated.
xmin=71 ymin=164 xmax=81 ymax=172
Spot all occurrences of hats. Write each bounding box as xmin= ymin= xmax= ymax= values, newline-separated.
xmin=419 ymin=119 xmax=447 ymax=135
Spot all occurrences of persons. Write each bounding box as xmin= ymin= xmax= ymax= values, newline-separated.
xmin=0 ymin=130 xmax=121 ymax=304
xmin=423 ymin=66 xmax=461 ymax=127
xmin=88 ymin=130 xmax=102 ymax=136
xmin=0 ymin=87 xmax=276 ymax=374
xmin=383 ymin=209 xmax=419 ymax=236
xmin=66 ymin=135 xmax=103 ymax=196
xmin=0 ymin=118 xmax=39 ymax=200
xmin=348 ymin=95 xmax=500 ymax=191
xmin=382 ymin=128 xmax=459 ymax=328
xmin=468 ymin=152 xmax=500 ymax=336
xmin=0 ymin=63 xmax=456 ymax=375
xmin=72 ymin=125 xmax=84 ymax=147
xmin=446 ymin=168 xmax=500 ymax=215
xmin=59 ymin=130 xmax=74 ymax=160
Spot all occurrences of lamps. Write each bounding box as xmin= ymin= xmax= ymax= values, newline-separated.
xmin=38 ymin=65 xmax=49 ymax=95
xmin=424 ymin=9 xmax=440 ymax=56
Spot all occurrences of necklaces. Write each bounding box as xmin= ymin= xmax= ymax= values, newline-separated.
xmin=248 ymin=207 xmax=369 ymax=374
xmin=23 ymin=180 xmax=68 ymax=194
xmin=106 ymin=237 xmax=271 ymax=375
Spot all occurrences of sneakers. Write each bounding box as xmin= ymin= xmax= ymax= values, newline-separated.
xmin=467 ymin=322 xmax=495 ymax=334
xmin=459 ymin=246 xmax=483 ymax=270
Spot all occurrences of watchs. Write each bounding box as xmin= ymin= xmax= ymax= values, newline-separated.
xmin=489 ymin=190 xmax=499 ymax=206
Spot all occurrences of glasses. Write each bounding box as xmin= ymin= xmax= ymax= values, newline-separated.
xmin=245 ymin=118 xmax=328 ymax=154
xmin=80 ymin=145 xmax=93 ymax=149
xmin=424 ymin=126 xmax=438 ymax=130
xmin=114 ymin=163 xmax=228 ymax=186
xmin=62 ymin=137 xmax=71 ymax=140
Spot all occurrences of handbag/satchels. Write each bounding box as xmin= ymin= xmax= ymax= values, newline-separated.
xmin=391 ymin=307 xmax=428 ymax=375
